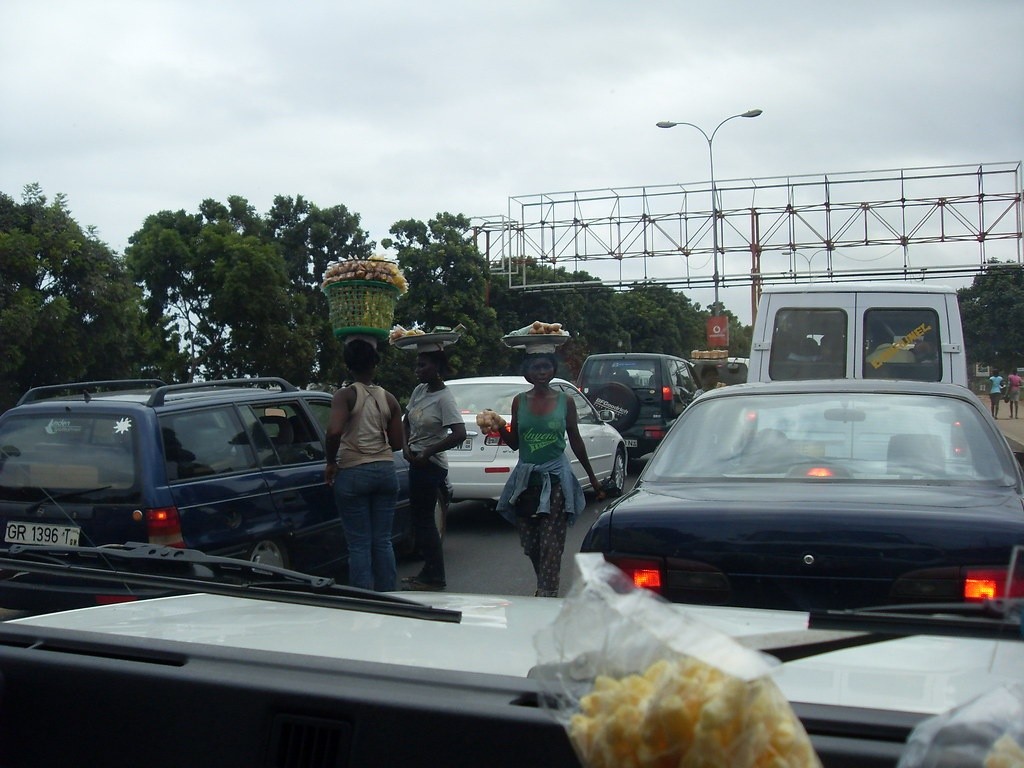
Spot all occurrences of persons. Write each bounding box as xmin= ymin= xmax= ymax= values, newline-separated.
xmin=988 ymin=369 xmax=1006 ymax=420
xmin=866 ymin=321 xmax=916 ymax=363
xmin=693 ymin=365 xmax=728 ymax=400
xmin=1004 ymin=369 xmax=1023 ymax=419
xmin=399 ymin=352 xmax=467 ymax=593
xmin=323 ymin=340 xmax=401 ymax=592
xmin=485 ymin=353 xmax=604 ymax=597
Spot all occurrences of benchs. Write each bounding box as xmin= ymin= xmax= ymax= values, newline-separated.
xmin=23 ymin=441 xmax=133 ymax=483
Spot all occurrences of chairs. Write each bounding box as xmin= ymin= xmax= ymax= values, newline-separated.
xmin=740 ymin=428 xmax=793 ymax=470
xmin=258 ymin=417 xmax=295 ymax=446
xmin=885 ymin=432 xmax=945 ymax=479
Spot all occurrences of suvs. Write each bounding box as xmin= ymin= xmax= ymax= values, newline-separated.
xmin=576 ymin=353 xmax=750 ymax=460
xmin=0 ymin=377 xmax=454 ymax=611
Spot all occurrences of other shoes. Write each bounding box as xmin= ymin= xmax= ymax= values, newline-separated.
xmin=400 ymin=576 xmax=443 ymax=591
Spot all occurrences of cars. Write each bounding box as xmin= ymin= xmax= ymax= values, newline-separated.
xmin=401 ymin=376 xmax=628 ymax=505
xmin=580 ymin=378 xmax=1024 ymax=611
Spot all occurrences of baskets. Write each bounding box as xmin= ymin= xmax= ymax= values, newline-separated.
xmin=322 ymin=281 xmax=400 ymax=343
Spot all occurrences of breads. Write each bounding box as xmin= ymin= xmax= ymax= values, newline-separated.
xmin=528 ymin=320 xmax=564 ymax=335
xmin=477 ymin=410 xmax=506 ymax=434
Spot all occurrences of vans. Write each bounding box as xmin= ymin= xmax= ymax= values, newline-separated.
xmin=747 ymin=282 xmax=968 ymax=388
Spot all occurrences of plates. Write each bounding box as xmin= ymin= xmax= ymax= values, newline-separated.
xmin=394 ymin=333 xmax=458 ymax=349
xmin=503 ymin=335 xmax=570 ymax=349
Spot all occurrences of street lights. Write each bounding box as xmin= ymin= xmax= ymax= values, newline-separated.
xmin=656 ymin=110 xmax=762 ymax=350
